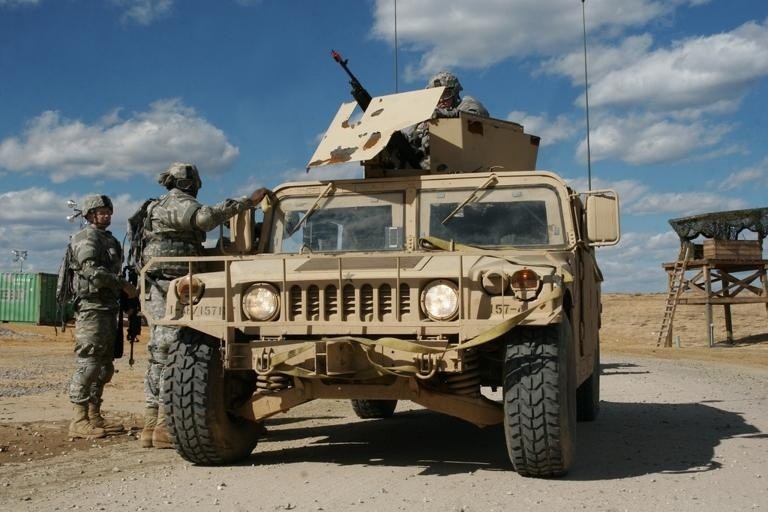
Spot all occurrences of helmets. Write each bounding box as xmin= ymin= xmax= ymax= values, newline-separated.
xmin=428 ymin=73 xmax=463 ymax=90
xmin=81 ymin=195 xmax=113 ymax=216
xmin=169 ymin=162 xmax=202 ymax=191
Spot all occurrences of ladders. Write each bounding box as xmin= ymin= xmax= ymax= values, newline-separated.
xmin=656 ymin=247 xmax=691 ymax=348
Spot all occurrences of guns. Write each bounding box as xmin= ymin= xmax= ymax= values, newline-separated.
xmin=121 ymin=265 xmax=142 ymax=365
xmin=333 ymin=51 xmax=418 ymax=168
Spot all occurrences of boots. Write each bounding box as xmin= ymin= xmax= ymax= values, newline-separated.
xmin=152 ymin=405 xmax=173 ymax=450
xmin=88 ymin=402 xmax=125 ymax=432
xmin=141 ymin=408 xmax=157 ymax=449
xmin=68 ymin=404 xmax=105 ymax=439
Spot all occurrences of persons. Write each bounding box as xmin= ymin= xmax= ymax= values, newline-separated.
xmin=140 ymin=161 xmax=268 ymax=448
xmin=67 ymin=195 xmax=138 ymax=439
xmin=408 ymin=71 xmax=489 ymax=171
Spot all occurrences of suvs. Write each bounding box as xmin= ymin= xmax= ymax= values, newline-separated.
xmin=140 ymin=86 xmax=620 ymax=478
